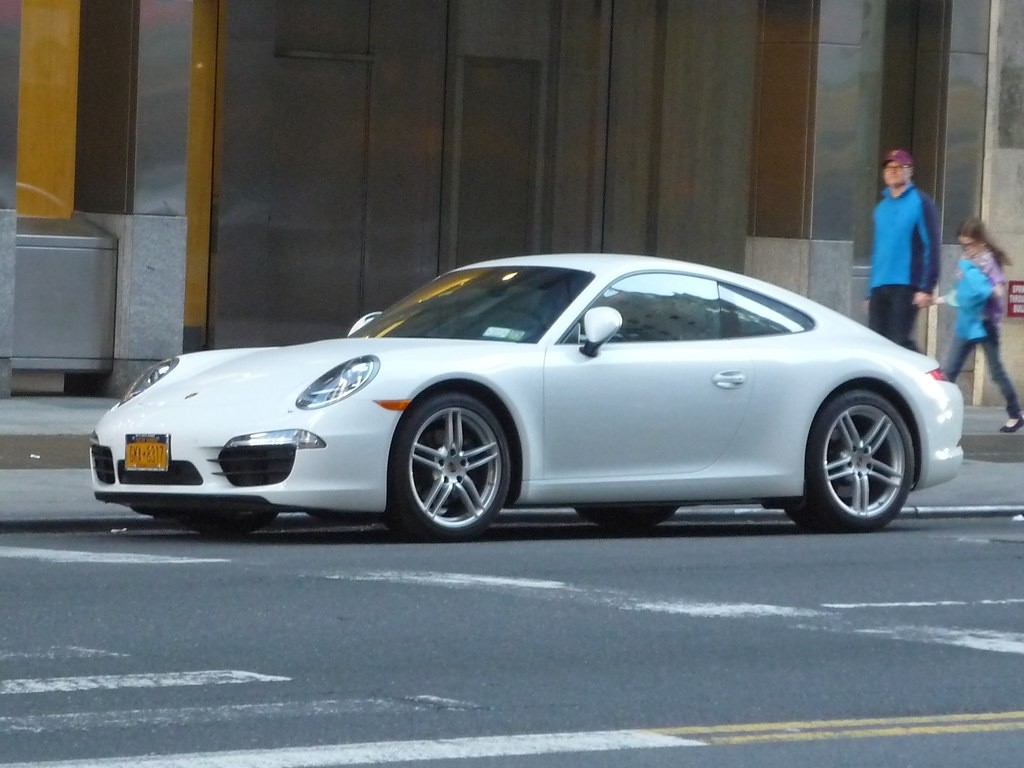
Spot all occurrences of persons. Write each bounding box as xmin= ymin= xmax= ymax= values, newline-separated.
xmin=864 ymin=150 xmax=941 ymax=352
xmin=930 ymin=220 xmax=1024 ymax=433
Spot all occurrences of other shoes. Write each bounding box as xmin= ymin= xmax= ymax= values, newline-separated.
xmin=1000 ymin=417 xmax=1024 ymax=432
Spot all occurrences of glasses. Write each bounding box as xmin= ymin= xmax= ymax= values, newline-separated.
xmin=882 ymin=164 xmax=912 ymax=171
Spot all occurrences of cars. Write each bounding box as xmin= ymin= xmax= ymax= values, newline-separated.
xmin=88 ymin=251 xmax=969 ymax=534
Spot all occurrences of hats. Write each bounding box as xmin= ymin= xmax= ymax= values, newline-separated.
xmin=880 ymin=149 xmax=914 ymax=168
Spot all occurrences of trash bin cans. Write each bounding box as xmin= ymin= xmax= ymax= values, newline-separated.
xmin=12 ymin=215 xmax=117 ymax=374
